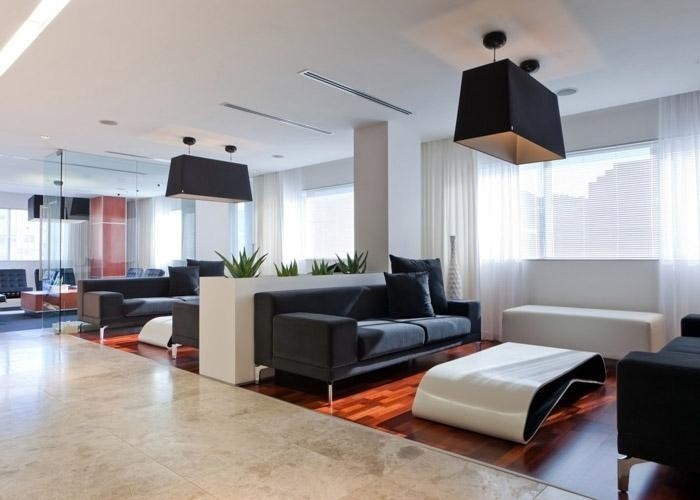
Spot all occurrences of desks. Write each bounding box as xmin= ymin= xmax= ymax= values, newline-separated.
xmin=0 ymin=294 xmax=7 ymax=303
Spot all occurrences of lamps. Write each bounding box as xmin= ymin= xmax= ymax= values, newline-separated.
xmin=449 ymin=30 xmax=567 ymax=168
xmin=27 ymin=180 xmax=91 ymax=224
xmin=164 ymin=136 xmax=253 ymax=204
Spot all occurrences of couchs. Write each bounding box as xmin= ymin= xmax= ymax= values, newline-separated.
xmin=171 ymin=297 xmax=201 ymax=361
xmin=499 ymin=302 xmax=667 ymax=360
xmin=0 ymin=269 xmax=33 ymax=297
xmin=251 ymin=282 xmax=483 ymax=407
xmin=613 ymin=312 xmax=700 ymax=495
xmin=77 ymin=277 xmax=200 ymax=344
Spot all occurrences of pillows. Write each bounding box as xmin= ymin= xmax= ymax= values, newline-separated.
xmin=169 ymin=265 xmax=200 ymax=298
xmin=388 ymin=253 xmax=450 ymax=316
xmin=383 ymin=269 xmax=435 ymax=319
xmin=187 ymin=258 xmax=226 ymax=276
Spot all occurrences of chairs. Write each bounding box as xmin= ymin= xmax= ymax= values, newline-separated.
xmin=125 ymin=267 xmax=143 ymax=277
xmin=144 ymin=268 xmax=165 ymax=277
xmin=34 ymin=267 xmax=75 ymax=290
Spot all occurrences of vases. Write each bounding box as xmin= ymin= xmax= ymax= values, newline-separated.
xmin=447 ymin=235 xmax=465 ymax=300
